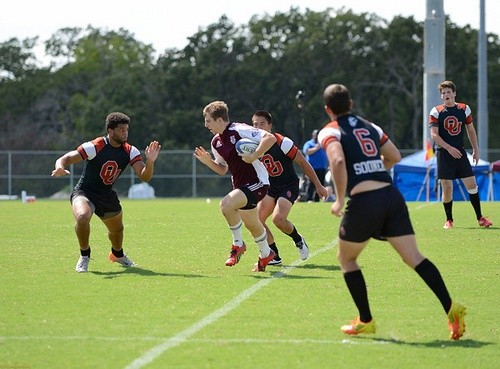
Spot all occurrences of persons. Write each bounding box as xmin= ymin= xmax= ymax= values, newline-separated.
xmin=51 ymin=112 xmax=161 ymax=272
xmin=194 ymin=101 xmax=277 ymax=272
xmin=251 ymin=111 xmax=328 ymax=265
xmin=294 ymin=172 xmax=337 ymax=203
xmin=317 ymin=84 xmax=467 ymax=340
xmin=303 ymin=129 xmax=330 ymax=203
xmin=429 ymin=81 xmax=493 ymax=229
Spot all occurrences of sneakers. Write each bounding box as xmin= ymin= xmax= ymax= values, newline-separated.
xmin=447 ymin=303 xmax=466 ymax=339
xmin=252 ymin=248 xmax=276 ymax=272
xmin=293 ymin=233 xmax=308 ymax=260
xmin=109 ymin=251 xmax=134 ymax=267
xmin=76 ymin=254 xmax=90 ymax=273
xmin=225 ymin=240 xmax=246 ymax=267
xmin=443 ymin=220 xmax=453 ymax=229
xmin=341 ymin=316 xmax=375 ymax=334
xmin=479 ymin=216 xmax=492 ymax=227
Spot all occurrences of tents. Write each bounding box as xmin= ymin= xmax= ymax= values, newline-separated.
xmin=391 ymin=146 xmax=500 ymax=202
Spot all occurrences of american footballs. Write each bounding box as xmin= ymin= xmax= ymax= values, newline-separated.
xmin=236 ymin=137 xmax=264 ymax=160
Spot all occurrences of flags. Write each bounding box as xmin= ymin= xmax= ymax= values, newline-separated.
xmin=425 ymin=139 xmax=434 ymax=161
